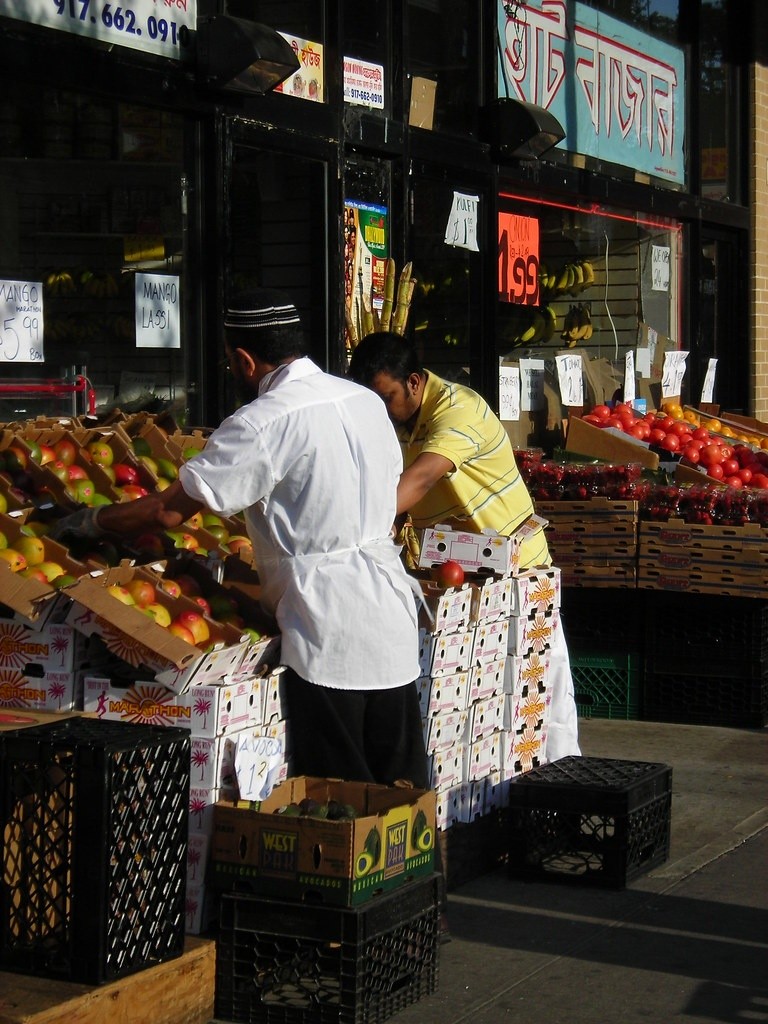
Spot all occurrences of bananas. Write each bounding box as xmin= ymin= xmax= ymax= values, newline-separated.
xmin=413 ymin=268 xmax=462 ymax=346
xmin=509 ymin=258 xmax=594 ymax=348
xmin=39 ymin=265 xmax=143 ymax=340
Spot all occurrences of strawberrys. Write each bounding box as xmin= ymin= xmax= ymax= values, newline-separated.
xmin=642 ymin=484 xmax=768 ymax=529
xmin=511 ymin=449 xmax=651 ymax=503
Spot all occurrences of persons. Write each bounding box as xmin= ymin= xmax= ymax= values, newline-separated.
xmin=349 ymin=333 xmax=585 ymax=766
xmin=344 ymin=207 xmax=359 ymax=347
xmin=54 ymin=289 xmax=429 ymax=791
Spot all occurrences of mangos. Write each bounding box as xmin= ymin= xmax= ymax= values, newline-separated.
xmin=434 ymin=560 xmax=464 ymax=588
xmin=0 ymin=436 xmax=261 ymax=652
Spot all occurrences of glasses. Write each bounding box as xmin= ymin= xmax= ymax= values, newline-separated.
xmin=214 ymin=350 xmax=239 ymax=372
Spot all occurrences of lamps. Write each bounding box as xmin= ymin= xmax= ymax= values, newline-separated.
xmin=179 ymin=14 xmax=300 ymax=109
xmin=471 ymin=97 xmax=566 ymax=184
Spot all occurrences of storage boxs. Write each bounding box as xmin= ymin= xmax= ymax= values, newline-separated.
xmin=414 ymin=566 xmax=561 ymax=832
xmin=569 ymin=651 xmax=645 ymax=721
xmin=0 ymin=618 xmax=113 ymax=675
xmin=508 ymin=756 xmax=673 ymax=894
xmin=84 ymin=668 xmax=263 ymax=740
xmin=565 ymin=416 xmax=683 ymax=476
xmin=184 ymin=665 xmax=296 ymax=938
xmin=60 ymin=557 xmax=252 ymax=698
xmin=213 ymin=871 xmax=440 ymax=1024
xmin=682 ymin=404 xmax=768 ymax=452
xmin=530 ymin=496 xmax=768 ymax=600
xmin=561 ymin=587 xmax=647 ymax=650
xmin=676 ymin=435 xmax=768 ymax=487
xmin=0 ymin=408 xmax=272 ymax=643
xmin=212 ymin=776 xmax=436 ymax=908
xmin=0 ymin=512 xmax=108 ymax=633
xmin=0 ymin=715 xmax=192 ymax=988
xmin=643 ymin=674 xmax=768 ymax=729
xmin=0 ymin=667 xmax=114 ymax=714
xmin=418 ymin=514 xmax=550 ymax=580
xmin=644 ymin=590 xmax=768 ymax=679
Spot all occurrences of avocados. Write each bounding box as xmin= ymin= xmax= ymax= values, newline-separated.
xmin=354 ymin=826 xmax=381 ymax=879
xmin=411 ymin=809 xmax=433 ymax=852
xmin=271 ymin=796 xmax=357 ymax=822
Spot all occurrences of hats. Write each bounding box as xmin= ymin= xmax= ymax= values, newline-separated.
xmin=224 ymin=289 xmax=300 ymax=328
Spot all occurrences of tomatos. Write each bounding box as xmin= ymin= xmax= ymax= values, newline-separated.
xmin=581 ymin=404 xmax=768 ymax=490
